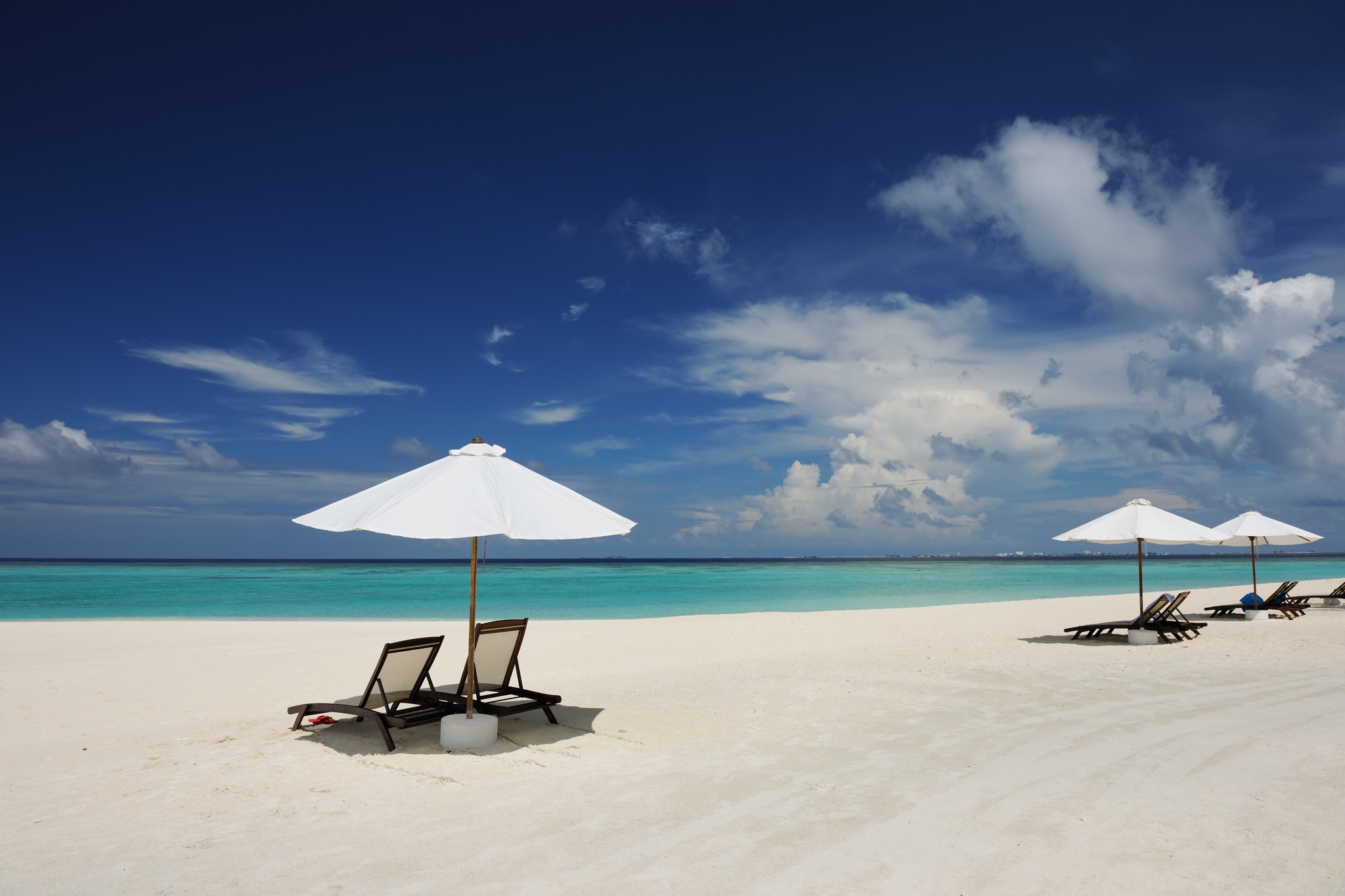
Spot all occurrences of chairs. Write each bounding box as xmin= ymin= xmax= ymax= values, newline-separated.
xmin=287 ymin=636 xmax=456 ymax=751
xmin=1064 ymin=595 xmax=1189 ymax=645
xmin=1204 ymin=581 xmax=1345 ymax=620
xmin=388 ymin=618 xmax=561 ymax=736
xmin=1093 ymin=592 xmax=1207 ymax=640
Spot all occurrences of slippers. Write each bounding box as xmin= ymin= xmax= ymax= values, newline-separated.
xmin=308 ymin=715 xmax=335 ymax=722
xmin=312 ymin=716 xmax=338 ymax=724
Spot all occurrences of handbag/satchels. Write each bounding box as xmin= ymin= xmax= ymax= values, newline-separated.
xmin=1239 ymin=592 xmax=1264 ymax=605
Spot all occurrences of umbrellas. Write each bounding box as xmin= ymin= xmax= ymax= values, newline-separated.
xmin=1209 ymin=510 xmax=1326 ymax=610
xmin=293 ymin=436 xmax=639 ymax=720
xmin=1054 ymin=499 xmax=1229 ymax=631
